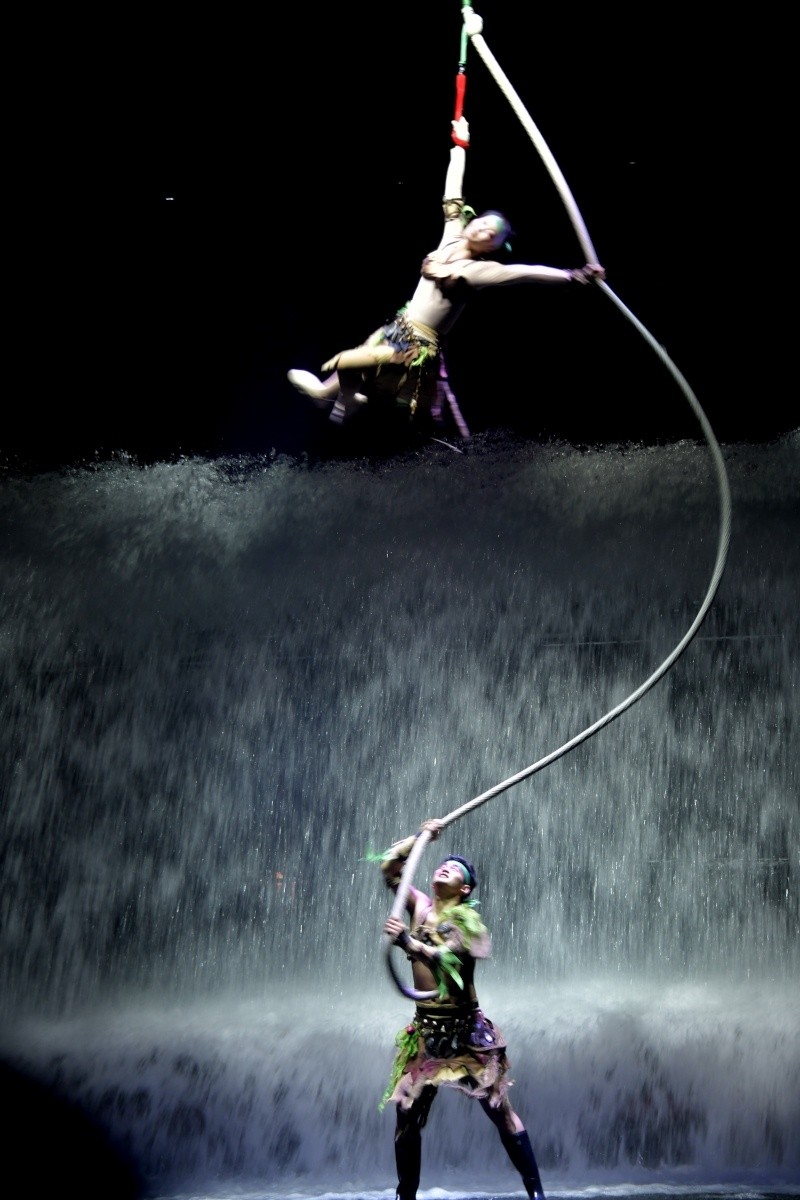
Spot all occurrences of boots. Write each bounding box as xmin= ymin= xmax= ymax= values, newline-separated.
xmin=500 ymin=1130 xmax=546 ymax=1200
xmin=394 ymin=1135 xmax=422 ymax=1200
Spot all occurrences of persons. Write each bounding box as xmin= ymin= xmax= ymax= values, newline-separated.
xmin=285 ymin=116 xmax=607 ymax=424
xmin=379 ymin=816 xmax=549 ymax=1200
